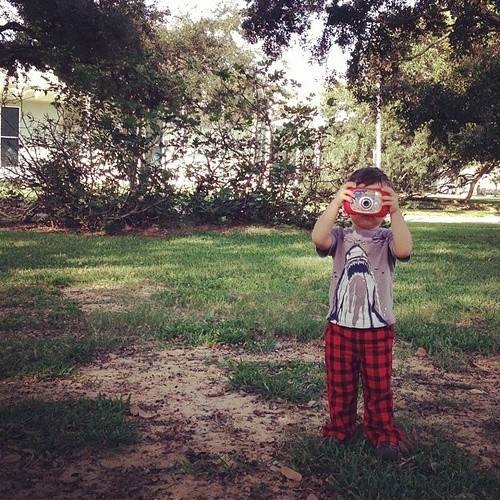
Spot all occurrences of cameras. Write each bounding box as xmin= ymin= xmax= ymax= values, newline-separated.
xmin=342 ymin=187 xmax=391 ymax=216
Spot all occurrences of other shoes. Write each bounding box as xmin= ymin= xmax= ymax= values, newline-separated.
xmin=321 ymin=437 xmax=342 ymax=447
xmin=374 ymin=441 xmax=400 ymax=461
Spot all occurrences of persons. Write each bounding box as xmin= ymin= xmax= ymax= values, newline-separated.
xmin=310 ymin=167 xmax=412 ymax=454
